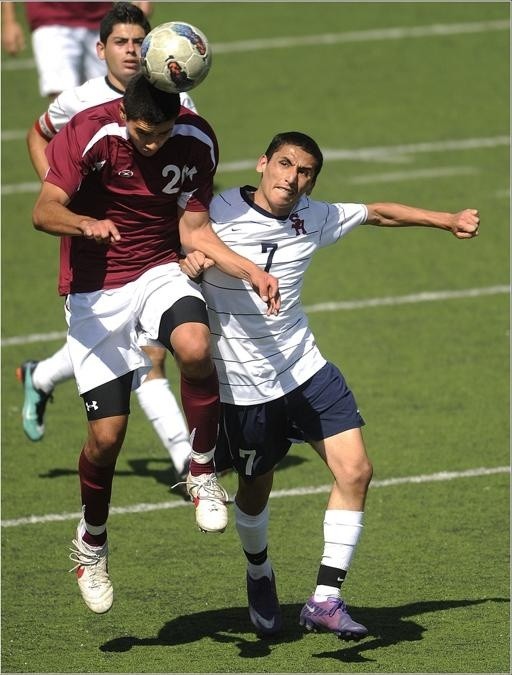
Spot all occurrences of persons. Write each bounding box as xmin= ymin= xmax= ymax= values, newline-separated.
xmin=15 ymin=4 xmax=233 ymax=506
xmin=2 ymin=1 xmax=152 ymax=105
xmin=31 ymin=73 xmax=280 ymax=615
xmin=179 ymin=131 xmax=481 ymax=639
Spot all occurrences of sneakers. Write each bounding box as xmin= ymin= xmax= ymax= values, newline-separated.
xmin=246 ymin=567 xmax=280 ymax=634
xmin=15 ymin=359 xmax=52 ymax=441
xmin=75 ymin=519 xmax=113 ymax=614
xmin=170 ymin=455 xmax=228 ymax=533
xmin=299 ymin=596 xmax=368 ymax=642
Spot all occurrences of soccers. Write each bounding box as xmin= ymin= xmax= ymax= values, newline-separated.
xmin=139 ymin=22 xmax=212 ymax=92
